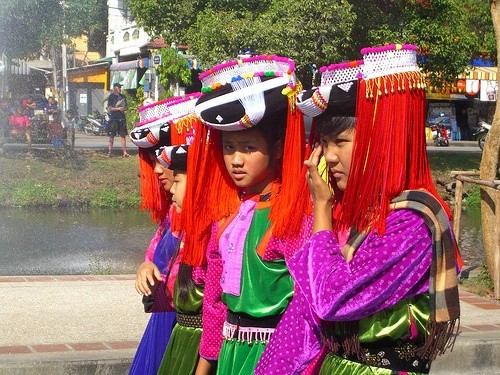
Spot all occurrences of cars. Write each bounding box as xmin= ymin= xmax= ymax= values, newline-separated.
xmin=11 ymin=92 xmax=119 ymax=144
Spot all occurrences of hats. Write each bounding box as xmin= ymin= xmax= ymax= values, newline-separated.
xmin=156 ymin=145 xmax=193 ymax=171
xmin=114 ymin=83 xmax=123 ymax=86
xmin=294 ymin=43 xmax=427 ymax=116
xmin=194 ymin=56 xmax=303 ymax=131
xmin=129 ymin=91 xmax=204 ymax=149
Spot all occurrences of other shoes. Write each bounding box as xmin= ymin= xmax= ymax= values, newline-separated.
xmin=106 ymin=152 xmax=113 ymax=158
xmin=123 ymin=153 xmax=131 ymax=157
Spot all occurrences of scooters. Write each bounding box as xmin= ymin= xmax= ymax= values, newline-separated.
xmin=473 ymin=120 xmax=492 ymax=150
xmin=428 ymin=112 xmax=450 ymax=148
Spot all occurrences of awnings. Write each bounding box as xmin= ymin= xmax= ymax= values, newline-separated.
xmin=415 ymin=65 xmax=498 ymax=94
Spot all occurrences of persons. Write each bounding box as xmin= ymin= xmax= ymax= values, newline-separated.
xmin=47 ymin=109 xmax=77 ymax=159
xmin=19 ymin=98 xmax=34 ymax=124
xmin=193 ymin=55 xmax=294 ymax=375
xmin=156 ymin=146 xmax=207 ymax=375
xmin=9 ymin=105 xmax=35 ymax=158
xmin=105 ymin=83 xmax=132 ymax=159
xmin=251 ymin=45 xmax=464 ymax=375
xmin=0 ymin=96 xmax=14 ymax=137
xmin=44 ymin=96 xmax=57 ymax=115
xmin=129 ymin=89 xmax=204 ymax=375
